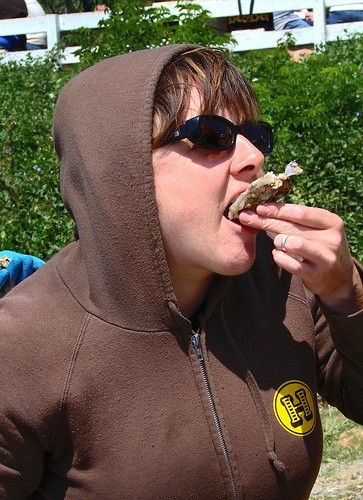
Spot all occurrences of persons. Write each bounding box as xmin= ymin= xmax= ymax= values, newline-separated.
xmin=26 ymin=33 xmax=46 ymax=48
xmin=272 ymin=11 xmax=313 ymax=30
xmin=328 ymin=4 xmax=363 ymax=24
xmin=1 ymin=44 xmax=363 ymax=498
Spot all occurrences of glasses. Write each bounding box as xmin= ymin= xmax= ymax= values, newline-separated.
xmin=151 ymin=115 xmax=274 ymax=154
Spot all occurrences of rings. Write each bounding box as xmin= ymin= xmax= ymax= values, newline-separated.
xmin=281 ymin=235 xmax=288 ymax=253
